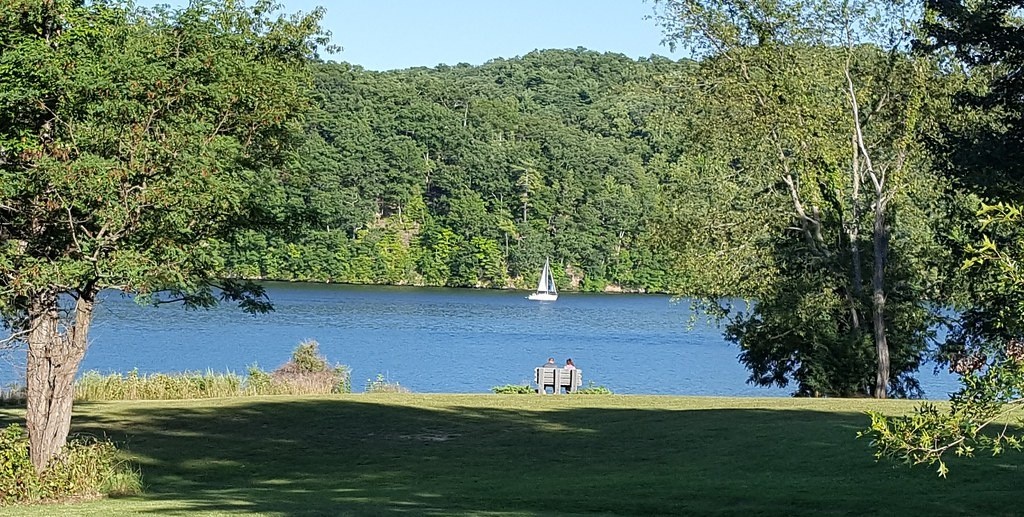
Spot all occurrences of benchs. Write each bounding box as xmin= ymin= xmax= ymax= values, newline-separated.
xmin=534 ymin=367 xmax=582 ymax=394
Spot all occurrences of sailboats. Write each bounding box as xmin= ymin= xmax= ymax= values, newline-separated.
xmin=528 ymin=254 xmax=560 ymax=301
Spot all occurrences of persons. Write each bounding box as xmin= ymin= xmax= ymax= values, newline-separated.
xmin=535 ymin=358 xmax=557 ymax=394
xmin=564 ymin=359 xmax=577 ymax=394
